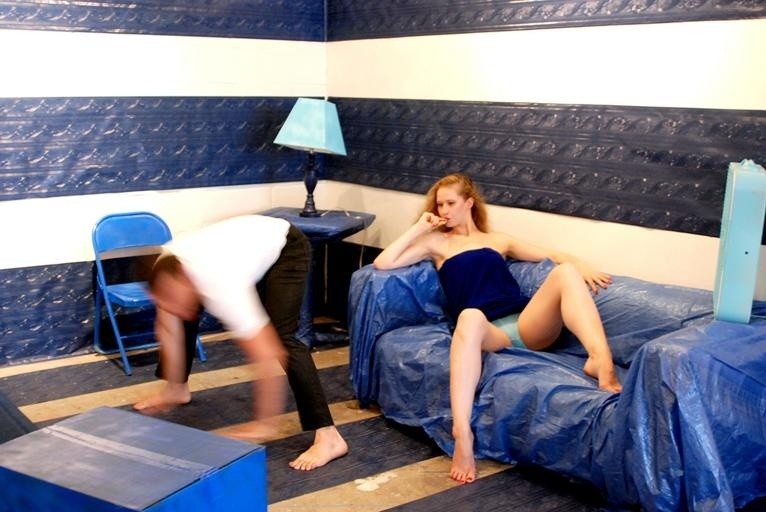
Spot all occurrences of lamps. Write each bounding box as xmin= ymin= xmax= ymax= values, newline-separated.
xmin=272 ymin=98 xmax=347 ymax=218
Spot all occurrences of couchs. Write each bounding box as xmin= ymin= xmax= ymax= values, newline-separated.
xmin=349 ymin=260 xmax=766 ymax=512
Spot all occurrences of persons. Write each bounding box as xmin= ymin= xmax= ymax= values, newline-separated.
xmin=131 ymin=210 xmax=350 ymax=472
xmin=371 ymin=172 xmax=627 ymax=485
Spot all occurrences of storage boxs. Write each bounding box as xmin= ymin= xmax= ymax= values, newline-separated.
xmin=0 ymin=405 xmax=268 ymax=510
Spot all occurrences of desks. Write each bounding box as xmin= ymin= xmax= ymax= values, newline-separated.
xmin=259 ymin=206 xmax=376 ymax=305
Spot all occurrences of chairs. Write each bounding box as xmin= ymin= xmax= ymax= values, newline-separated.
xmin=92 ymin=211 xmax=206 ymax=376
